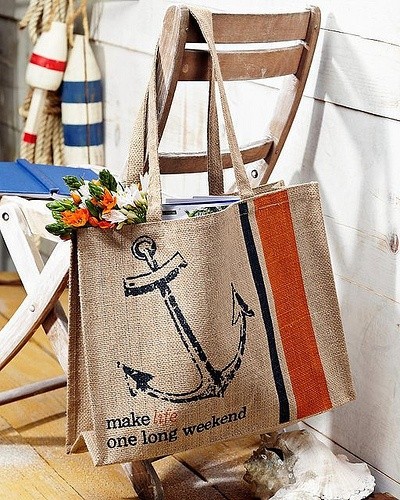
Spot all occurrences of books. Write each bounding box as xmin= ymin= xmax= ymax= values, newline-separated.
xmin=1 ymin=157 xmax=99 ymax=202
xmin=161 ymin=191 xmax=242 ymax=226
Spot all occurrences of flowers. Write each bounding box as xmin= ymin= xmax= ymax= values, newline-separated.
xmin=43 ymin=169 xmax=151 ymax=232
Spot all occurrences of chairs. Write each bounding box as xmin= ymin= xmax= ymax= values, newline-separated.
xmin=0 ymin=5 xmax=322 ymax=499
